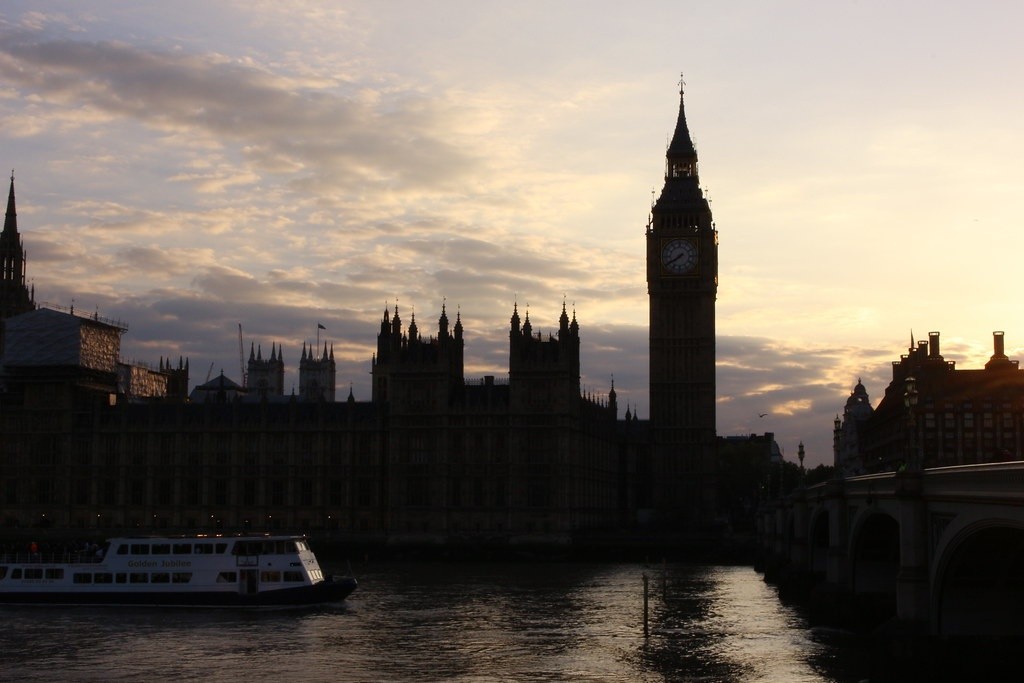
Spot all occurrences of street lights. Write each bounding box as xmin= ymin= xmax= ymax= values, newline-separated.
xmin=97 ymin=514 xmax=101 ymax=527
xmin=904 ymin=375 xmax=921 ymax=469
xmin=268 ymin=515 xmax=273 ymax=533
xmin=42 ymin=514 xmax=46 ymax=527
xmin=798 ymin=443 xmax=805 ymax=488
xmin=833 ymin=416 xmax=842 ymax=478
xmin=328 ymin=515 xmax=331 ymax=530
xmin=154 ymin=514 xmax=157 ymax=529
xmin=210 ymin=514 xmax=214 ymax=535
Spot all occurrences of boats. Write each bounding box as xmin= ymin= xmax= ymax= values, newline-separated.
xmin=0 ymin=537 xmax=360 ymax=610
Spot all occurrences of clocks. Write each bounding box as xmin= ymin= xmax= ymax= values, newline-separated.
xmin=660 ymin=237 xmax=699 ymax=276
